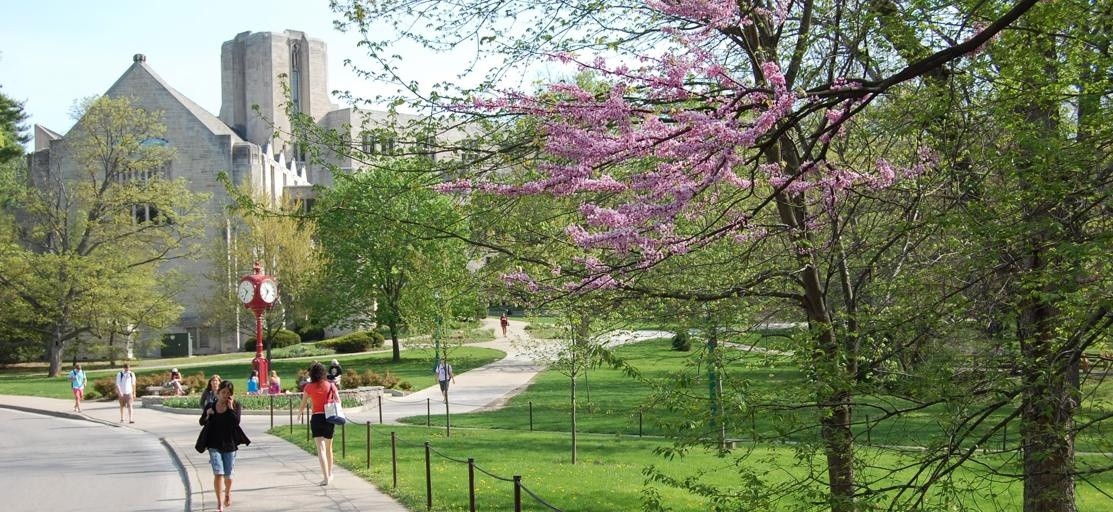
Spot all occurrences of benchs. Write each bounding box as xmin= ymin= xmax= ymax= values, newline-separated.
xmin=145 ymin=385 xmax=189 ymax=396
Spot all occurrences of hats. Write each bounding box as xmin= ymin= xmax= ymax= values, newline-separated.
xmin=172 ymin=369 xmax=178 ymax=374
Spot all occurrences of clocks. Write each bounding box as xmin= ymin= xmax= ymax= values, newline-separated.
xmin=259 ymin=279 xmax=277 ymax=303
xmin=237 ymin=276 xmax=257 ymax=304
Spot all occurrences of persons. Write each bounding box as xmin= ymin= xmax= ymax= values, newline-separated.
xmin=267 ymin=370 xmax=281 ymax=394
xmin=67 ymin=363 xmax=87 ymax=413
xmin=247 ymin=370 xmax=263 ymax=395
xmin=500 ymin=312 xmax=509 ymax=338
xmin=435 ymin=358 xmax=456 ymax=404
xmin=169 ymin=368 xmax=186 ymax=396
xmin=194 ymin=380 xmax=251 ymax=512
xmin=294 ymin=358 xmax=343 ymax=393
xmin=114 ymin=363 xmax=136 ymax=423
xmin=296 ymin=362 xmax=341 ymax=487
xmin=198 ymin=375 xmax=223 ymax=411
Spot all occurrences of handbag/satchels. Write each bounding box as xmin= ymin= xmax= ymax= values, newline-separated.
xmin=325 ymin=403 xmax=346 ymax=425
xmin=196 ymin=426 xmax=210 ymax=453
